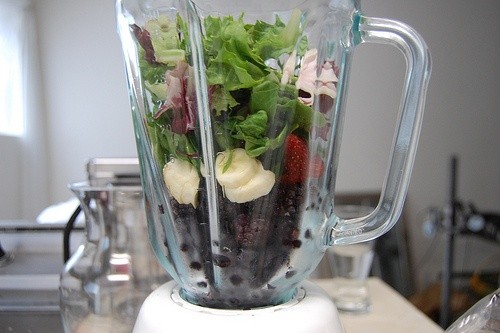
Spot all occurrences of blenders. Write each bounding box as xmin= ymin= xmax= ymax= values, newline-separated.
xmin=112 ymin=1 xmax=432 ymax=332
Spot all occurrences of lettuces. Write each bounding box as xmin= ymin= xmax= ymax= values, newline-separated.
xmin=128 ymin=10 xmax=327 ymax=172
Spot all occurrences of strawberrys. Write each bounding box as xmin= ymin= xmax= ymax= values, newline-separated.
xmin=273 ymin=133 xmax=325 ymax=187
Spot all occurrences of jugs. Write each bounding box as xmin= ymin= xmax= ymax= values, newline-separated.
xmin=57 ymin=155 xmax=168 ymax=333
xmin=322 ymin=204 xmax=382 ymax=312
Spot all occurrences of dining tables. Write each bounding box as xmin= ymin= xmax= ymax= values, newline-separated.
xmin=72 ymin=274 xmax=446 ymax=333
